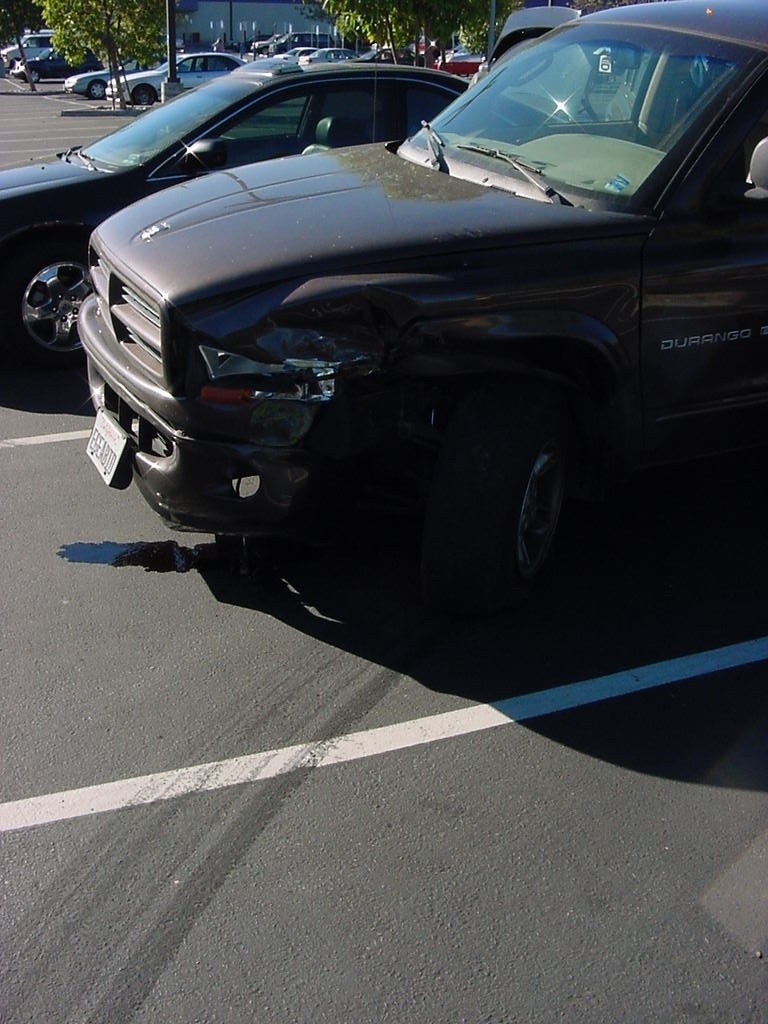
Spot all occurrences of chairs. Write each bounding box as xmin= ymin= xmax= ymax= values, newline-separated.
xmin=302 ymin=116 xmax=339 ymax=155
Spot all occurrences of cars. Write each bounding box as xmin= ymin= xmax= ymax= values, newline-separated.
xmin=105 ymin=51 xmax=249 ymax=107
xmin=12 ymin=45 xmax=107 ymax=84
xmin=0 ymin=63 xmax=572 ymax=376
xmin=0 ymin=29 xmax=63 ymax=69
xmin=62 ymin=52 xmax=168 ymax=100
xmin=231 ymin=30 xmax=496 ymax=78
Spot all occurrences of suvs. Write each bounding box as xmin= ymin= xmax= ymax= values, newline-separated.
xmin=78 ymin=0 xmax=768 ymax=624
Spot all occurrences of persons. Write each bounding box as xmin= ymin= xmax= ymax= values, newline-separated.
xmin=212 ymin=37 xmax=224 ymax=52
